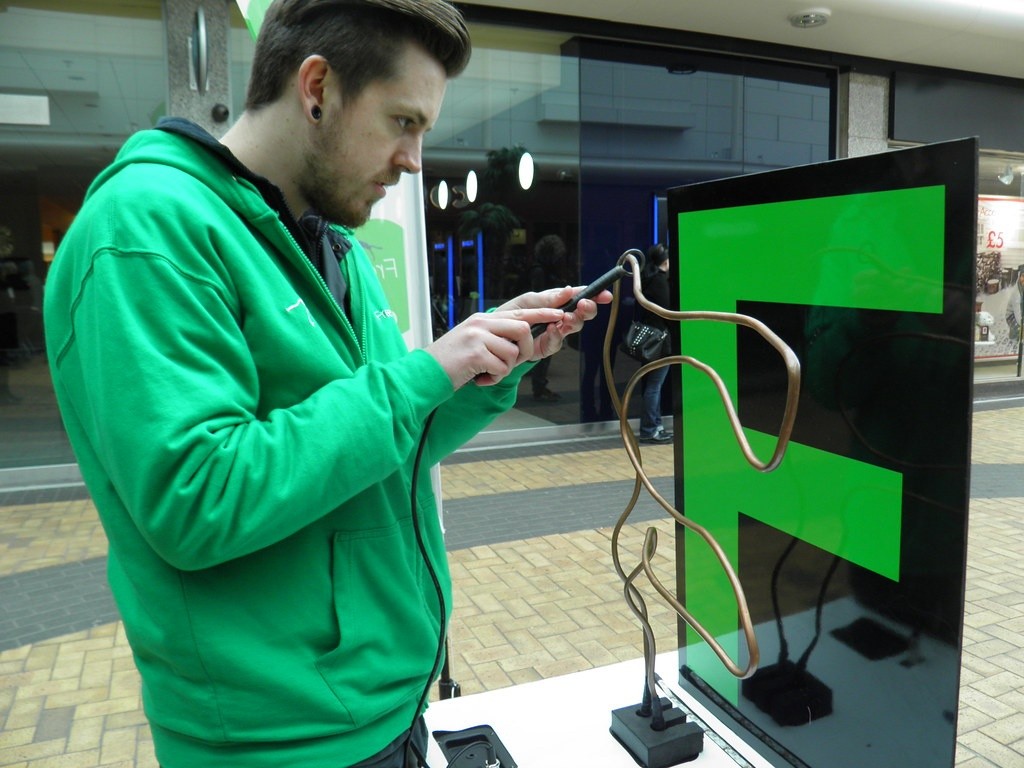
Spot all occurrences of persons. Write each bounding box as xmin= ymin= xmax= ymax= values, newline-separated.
xmin=41 ymin=0 xmax=611 ymax=768
xmin=1006 ymin=265 xmax=1024 ymax=339
xmin=527 ymin=234 xmax=571 ymax=404
xmin=634 ymin=243 xmax=675 ymax=444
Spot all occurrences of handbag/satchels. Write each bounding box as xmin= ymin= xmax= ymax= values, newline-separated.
xmin=618 ymin=320 xmax=673 ymax=363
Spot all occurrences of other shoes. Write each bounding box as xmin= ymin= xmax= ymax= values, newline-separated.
xmin=639 ymin=430 xmax=674 ymax=444
